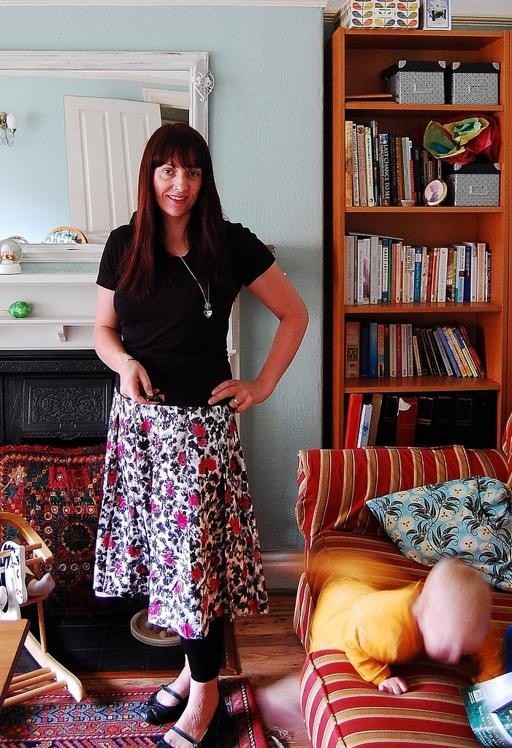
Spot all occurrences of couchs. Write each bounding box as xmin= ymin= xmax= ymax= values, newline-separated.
xmin=287 ymin=399 xmax=512 ymax=748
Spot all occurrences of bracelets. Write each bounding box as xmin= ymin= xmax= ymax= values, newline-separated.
xmin=120 ymin=352 xmax=135 ymax=363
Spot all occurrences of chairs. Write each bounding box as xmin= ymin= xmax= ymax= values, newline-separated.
xmin=2 ymin=506 xmax=85 ymax=712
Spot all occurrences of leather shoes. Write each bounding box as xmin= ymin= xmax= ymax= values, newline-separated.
xmin=151 ymin=694 xmax=230 ymax=748
xmin=138 ymin=677 xmax=188 ymax=724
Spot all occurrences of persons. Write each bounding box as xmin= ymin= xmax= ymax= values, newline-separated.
xmin=310 ymin=550 xmax=505 ymax=699
xmin=93 ymin=121 xmax=311 ymax=746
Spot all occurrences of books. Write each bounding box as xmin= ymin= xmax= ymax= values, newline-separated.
xmin=461 ymin=669 xmax=511 ymax=748
xmin=344 ymin=393 xmax=496 ymax=450
xmin=344 ymin=119 xmax=441 ymax=207
xmin=343 ymin=232 xmax=492 ymax=305
xmin=345 ymin=321 xmax=485 ymax=379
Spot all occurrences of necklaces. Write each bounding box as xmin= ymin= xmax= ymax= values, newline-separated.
xmin=168 ymin=230 xmax=213 ymax=319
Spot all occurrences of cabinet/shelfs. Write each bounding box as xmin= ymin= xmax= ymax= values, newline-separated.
xmin=0 ymin=348 xmax=120 ymax=671
xmin=326 ymin=26 xmax=510 ymax=453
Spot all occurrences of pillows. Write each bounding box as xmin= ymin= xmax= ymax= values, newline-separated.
xmin=362 ymin=473 xmax=512 ymax=592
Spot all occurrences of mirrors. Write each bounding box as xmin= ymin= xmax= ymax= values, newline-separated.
xmin=0 ymin=47 xmax=213 ymax=269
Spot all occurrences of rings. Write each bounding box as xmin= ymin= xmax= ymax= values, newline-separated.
xmin=232 ymin=396 xmax=241 ymax=406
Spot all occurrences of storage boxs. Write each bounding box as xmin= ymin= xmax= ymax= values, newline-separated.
xmin=377 ymin=56 xmax=446 ymax=105
xmin=439 ymin=153 xmax=499 ymax=211
xmin=334 ymin=1 xmax=421 ymax=33
xmin=448 ymin=52 xmax=500 ymax=107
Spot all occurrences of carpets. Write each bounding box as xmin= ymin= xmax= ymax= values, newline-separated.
xmin=4 ymin=671 xmax=269 ymax=747
